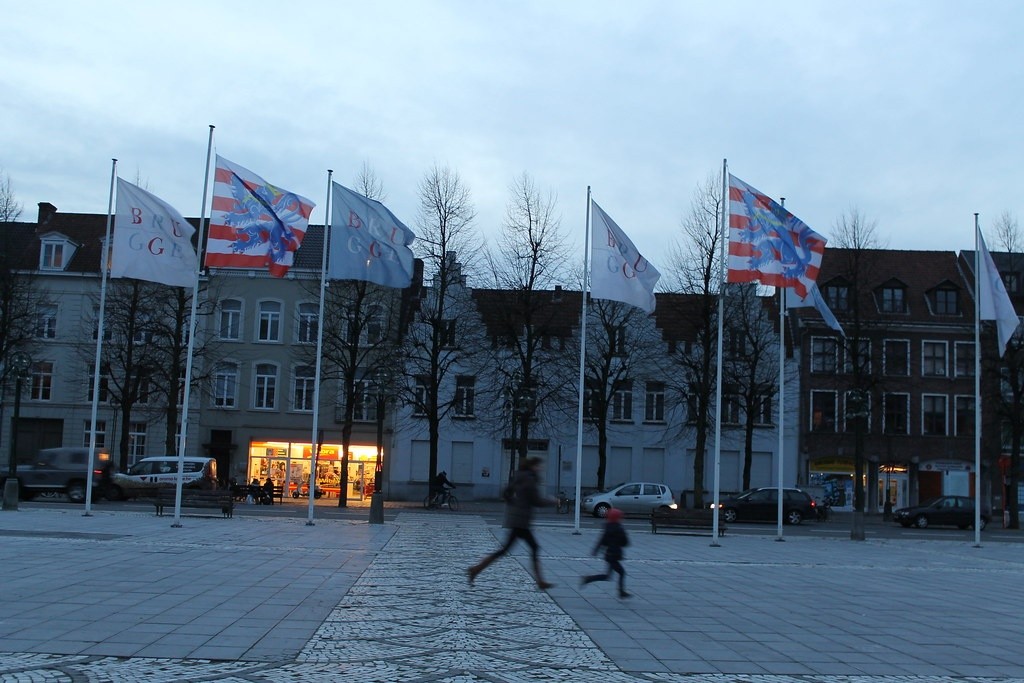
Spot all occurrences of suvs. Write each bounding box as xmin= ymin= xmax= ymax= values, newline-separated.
xmin=0 ymin=444 xmax=113 ymax=505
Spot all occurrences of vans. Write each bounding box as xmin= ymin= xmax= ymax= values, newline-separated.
xmin=108 ymin=455 xmax=219 ymax=495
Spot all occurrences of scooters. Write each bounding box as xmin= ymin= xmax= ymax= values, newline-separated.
xmin=291 ymin=479 xmax=325 ymax=500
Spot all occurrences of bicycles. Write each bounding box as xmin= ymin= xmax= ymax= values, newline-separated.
xmin=557 ymin=492 xmax=570 ymax=515
xmin=423 ymin=487 xmax=459 ymax=512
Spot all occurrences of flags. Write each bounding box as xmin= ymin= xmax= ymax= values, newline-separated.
xmin=786 ymin=281 xmax=846 ymax=338
xmin=979 ymin=229 xmax=1020 ymax=358
xmin=590 ymin=202 xmax=660 ymax=315
xmin=204 ymin=156 xmax=316 ymax=279
xmin=727 ymin=170 xmax=827 ymax=300
xmin=110 ymin=178 xmax=197 ymax=288
xmin=328 ymin=181 xmax=415 ymax=288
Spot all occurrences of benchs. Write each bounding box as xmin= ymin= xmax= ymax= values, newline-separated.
xmin=650 ymin=508 xmax=728 ymax=537
xmin=151 ymin=489 xmax=233 ymax=519
xmin=233 ymin=485 xmax=283 ymax=504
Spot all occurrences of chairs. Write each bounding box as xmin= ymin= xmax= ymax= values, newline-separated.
xmin=945 ymin=501 xmax=950 ymax=508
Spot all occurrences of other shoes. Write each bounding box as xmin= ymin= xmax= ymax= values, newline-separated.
xmin=619 ymin=591 xmax=632 ymax=599
xmin=467 ymin=567 xmax=477 ymax=589
xmin=536 ymin=581 xmax=555 ymax=589
xmin=579 ymin=575 xmax=586 ymax=589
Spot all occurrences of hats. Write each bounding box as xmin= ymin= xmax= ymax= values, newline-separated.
xmin=607 ymin=507 xmax=622 ymax=521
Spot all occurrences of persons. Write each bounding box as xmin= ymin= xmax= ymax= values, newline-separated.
xmin=468 ymin=456 xmax=553 ymax=589
xmin=260 ymin=478 xmax=274 ymax=505
xmin=433 ymin=471 xmax=456 ymax=507
xmin=580 ymin=508 xmax=631 ymax=597
xmin=250 ymin=479 xmax=261 ymax=504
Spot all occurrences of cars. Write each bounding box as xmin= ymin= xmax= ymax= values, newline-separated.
xmin=705 ymin=486 xmax=819 ymax=526
xmin=893 ymin=496 xmax=991 ymax=531
xmin=580 ymin=481 xmax=677 ymax=519
